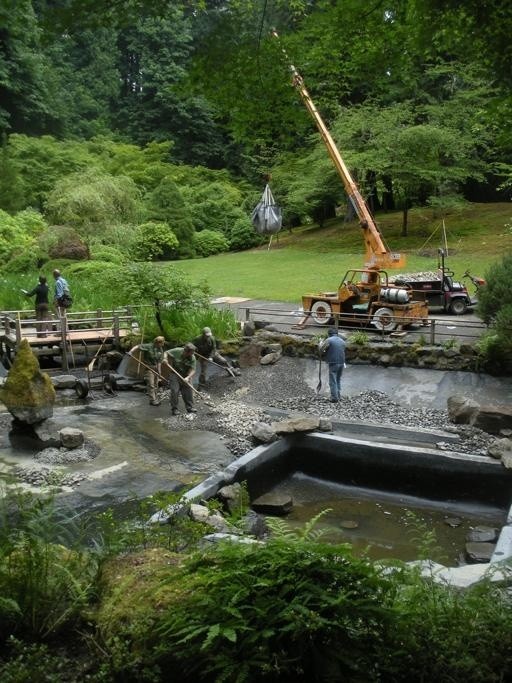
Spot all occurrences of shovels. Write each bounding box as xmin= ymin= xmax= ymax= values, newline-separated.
xmin=194 ymin=352 xmax=235 ymax=378
xmin=165 ymin=363 xmax=214 ymax=408
xmin=316 ymin=343 xmax=322 ymax=393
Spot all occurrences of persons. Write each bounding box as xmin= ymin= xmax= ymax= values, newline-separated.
xmin=163 ymin=343 xmax=197 ymax=415
xmin=21 ymin=275 xmax=50 ymax=338
xmin=52 ymin=269 xmax=69 ymax=337
xmin=128 ymin=336 xmax=165 ymax=406
xmin=318 ymin=326 xmax=347 ymax=403
xmin=191 ymin=327 xmax=242 ymax=393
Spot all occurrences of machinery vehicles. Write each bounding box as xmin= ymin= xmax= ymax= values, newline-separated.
xmin=268 ymin=25 xmax=430 ymax=339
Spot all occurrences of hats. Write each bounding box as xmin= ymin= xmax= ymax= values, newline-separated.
xmin=202 ymin=326 xmax=213 ymax=337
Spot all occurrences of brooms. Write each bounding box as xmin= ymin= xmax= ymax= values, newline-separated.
xmin=84 ymin=323 xmax=115 ymax=372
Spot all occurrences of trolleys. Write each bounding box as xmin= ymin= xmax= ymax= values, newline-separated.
xmin=76 ymin=335 xmax=120 ymax=403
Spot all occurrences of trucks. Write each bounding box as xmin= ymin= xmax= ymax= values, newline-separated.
xmin=386 ymin=247 xmax=488 ymax=316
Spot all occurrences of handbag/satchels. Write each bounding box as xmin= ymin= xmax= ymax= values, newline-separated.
xmin=55 ymin=281 xmax=73 ymax=309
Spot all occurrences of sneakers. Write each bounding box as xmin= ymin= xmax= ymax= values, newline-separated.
xmin=172 ymin=408 xmax=181 ymax=415
xmin=149 ymin=398 xmax=162 ymax=406
xmin=186 ymin=407 xmax=198 ymax=413
